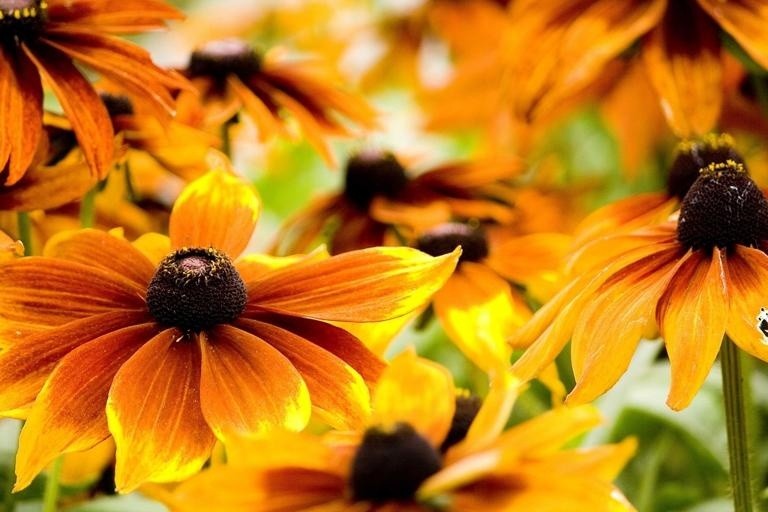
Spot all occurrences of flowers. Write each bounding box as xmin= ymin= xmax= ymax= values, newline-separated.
xmin=1 ymin=1 xmax=768 ymax=511
